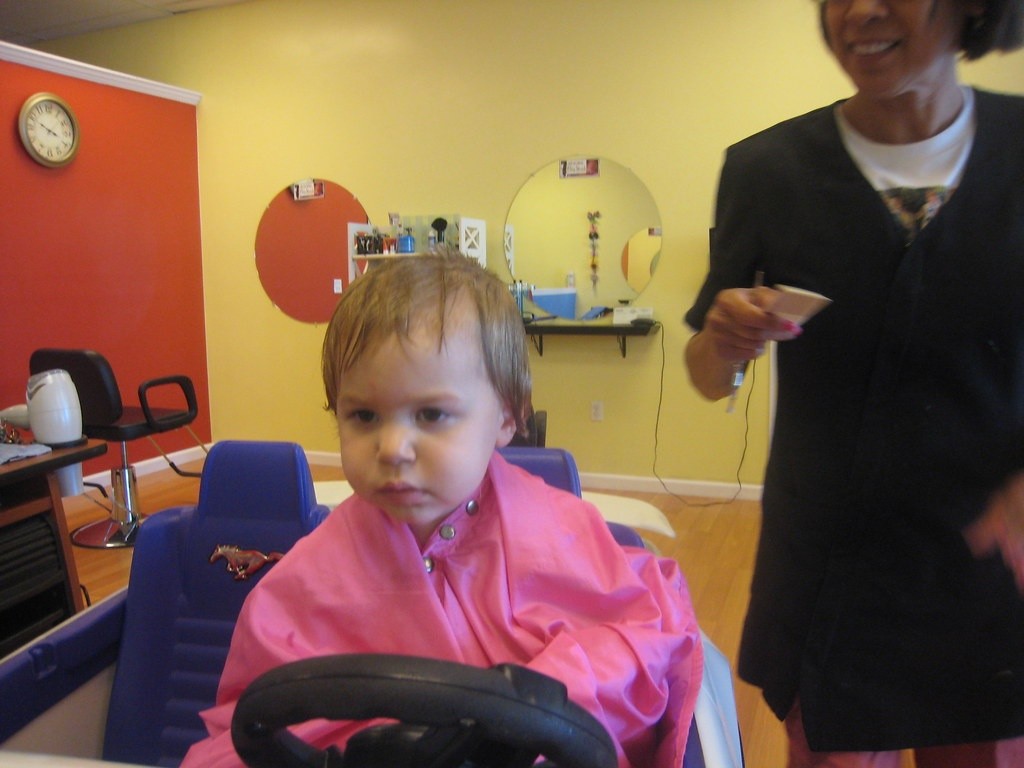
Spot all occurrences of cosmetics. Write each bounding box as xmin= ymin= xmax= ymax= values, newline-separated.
xmin=356 ymin=227 xmax=417 ymax=254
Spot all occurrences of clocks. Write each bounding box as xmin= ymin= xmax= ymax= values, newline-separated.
xmin=18 ymin=92 xmax=81 ymax=167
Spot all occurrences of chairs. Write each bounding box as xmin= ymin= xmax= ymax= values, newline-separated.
xmin=29 ymin=348 xmax=199 ymax=548
xmin=102 ymin=439 xmax=332 ymax=768
xmin=496 ymin=447 xmax=706 ymax=768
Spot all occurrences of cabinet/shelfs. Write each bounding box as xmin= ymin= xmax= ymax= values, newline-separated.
xmin=346 ymin=218 xmax=487 ymax=287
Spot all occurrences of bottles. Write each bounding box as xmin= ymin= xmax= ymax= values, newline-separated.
xmin=427 ymin=231 xmax=435 ymax=249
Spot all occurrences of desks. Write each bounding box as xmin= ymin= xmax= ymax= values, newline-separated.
xmin=0 ymin=422 xmax=108 ymax=619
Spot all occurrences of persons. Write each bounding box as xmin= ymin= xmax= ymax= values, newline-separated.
xmin=179 ymin=250 xmax=704 ymax=768
xmin=686 ymin=0 xmax=1024 ymax=767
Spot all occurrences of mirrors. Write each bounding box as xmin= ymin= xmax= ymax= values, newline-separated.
xmin=502 ymin=155 xmax=665 ymax=324
